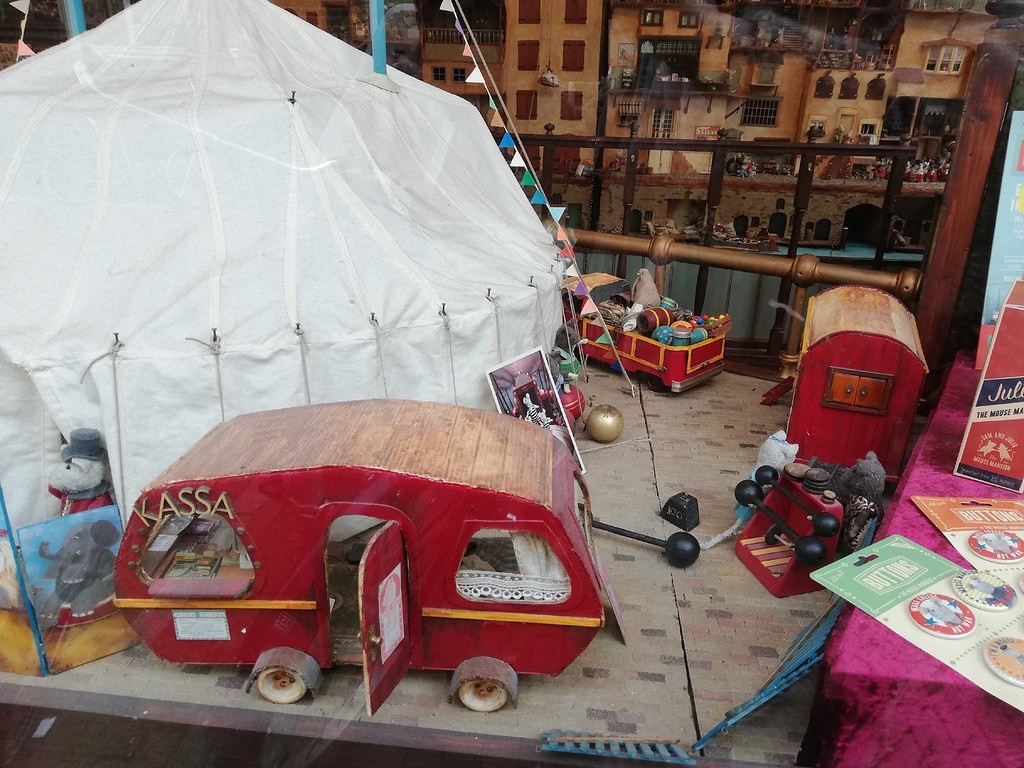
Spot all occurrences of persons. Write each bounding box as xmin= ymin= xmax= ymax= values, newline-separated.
xmin=876 ymin=156 xmax=937 ymax=182
xmin=833 ymin=130 xmax=853 ymax=144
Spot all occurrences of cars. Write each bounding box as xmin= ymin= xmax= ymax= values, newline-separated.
xmin=112 ymin=398 xmax=606 ymax=714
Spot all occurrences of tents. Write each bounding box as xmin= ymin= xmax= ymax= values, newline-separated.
xmin=0 ymin=0 xmax=564 ymax=548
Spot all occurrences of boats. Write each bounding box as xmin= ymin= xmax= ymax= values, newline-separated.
xmin=711 ymin=235 xmax=763 ymax=251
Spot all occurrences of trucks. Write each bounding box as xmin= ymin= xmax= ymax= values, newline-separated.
xmin=562 ymin=272 xmax=733 ymax=393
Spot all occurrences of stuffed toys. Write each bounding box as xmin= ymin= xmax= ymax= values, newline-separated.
xmin=46 ymin=428 xmax=113 ymax=516
